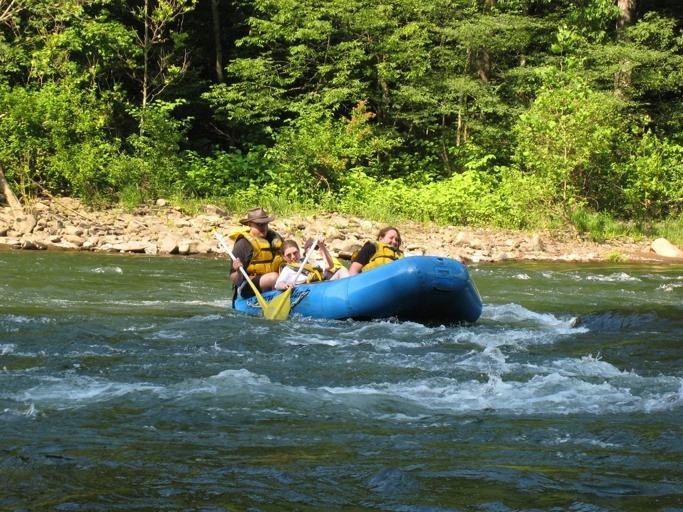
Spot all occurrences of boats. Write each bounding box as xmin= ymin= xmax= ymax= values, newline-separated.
xmin=231 ymin=256 xmax=481 ymax=324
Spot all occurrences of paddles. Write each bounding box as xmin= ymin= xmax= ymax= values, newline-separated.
xmin=215 ymin=232 xmax=268 ymax=312
xmin=264 ymin=232 xmax=321 ymax=321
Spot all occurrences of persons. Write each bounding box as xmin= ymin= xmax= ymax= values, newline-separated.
xmin=347 ymin=226 xmax=405 ymax=276
xmin=229 ymin=206 xmax=287 ymax=301
xmin=274 ymin=238 xmax=348 ymax=292
xmin=303 ymin=236 xmax=345 ymax=281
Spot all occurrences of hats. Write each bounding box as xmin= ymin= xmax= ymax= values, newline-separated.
xmin=303 ymin=239 xmax=326 ymax=256
xmin=239 ymin=207 xmax=273 ymax=224
